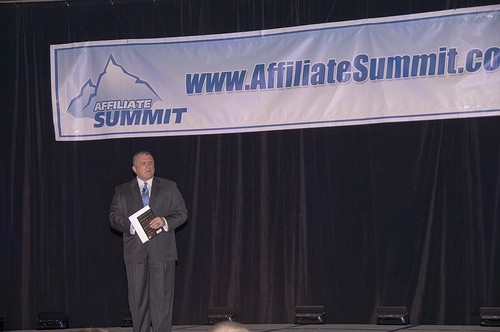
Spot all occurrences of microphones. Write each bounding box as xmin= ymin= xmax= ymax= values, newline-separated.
xmin=143 ymin=188 xmax=145 ymax=191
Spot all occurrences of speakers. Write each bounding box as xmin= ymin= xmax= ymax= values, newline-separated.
xmin=479 ymin=306 xmax=500 ymax=326
xmin=294 ymin=305 xmax=326 ymax=324
xmin=37 ymin=312 xmax=69 ymax=329
xmin=376 ymin=306 xmax=410 ymax=325
xmin=207 ymin=306 xmax=238 ymax=326
xmin=120 ymin=309 xmax=133 ymax=327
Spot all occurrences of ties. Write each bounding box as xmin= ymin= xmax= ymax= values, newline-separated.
xmin=142 ymin=182 xmax=150 ymax=207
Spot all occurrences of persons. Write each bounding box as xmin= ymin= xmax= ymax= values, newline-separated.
xmin=108 ymin=151 xmax=189 ymax=332
